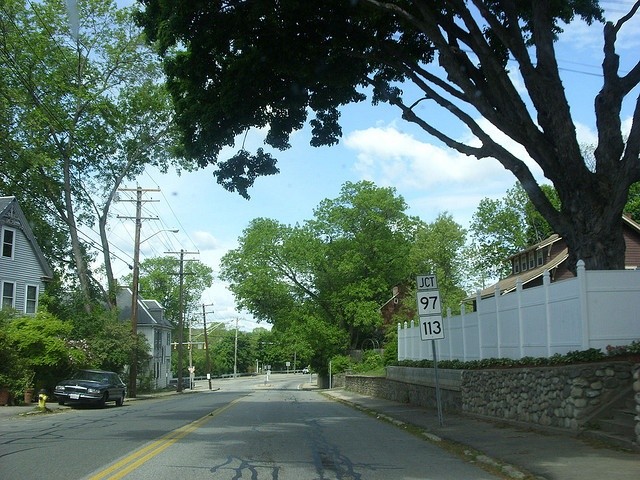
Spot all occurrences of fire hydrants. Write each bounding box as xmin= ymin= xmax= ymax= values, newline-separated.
xmin=37 ymin=388 xmax=48 ymax=410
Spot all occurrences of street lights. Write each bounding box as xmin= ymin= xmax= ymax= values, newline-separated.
xmin=129 ymin=229 xmax=181 ymax=399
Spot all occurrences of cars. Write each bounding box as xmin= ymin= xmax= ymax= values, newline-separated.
xmin=302 ymin=367 xmax=312 ymax=374
xmin=53 ymin=369 xmax=128 ymax=409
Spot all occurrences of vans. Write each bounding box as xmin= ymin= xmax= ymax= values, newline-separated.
xmin=170 ymin=378 xmax=195 ymax=389
